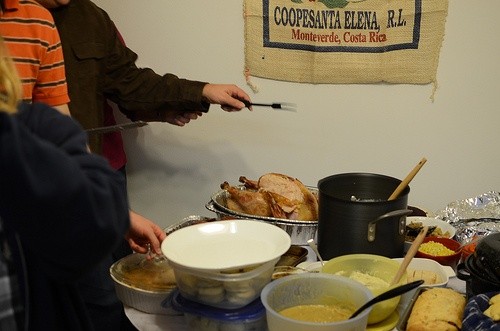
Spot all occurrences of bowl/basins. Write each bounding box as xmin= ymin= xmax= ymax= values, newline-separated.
xmin=414 ymin=237 xmax=463 ymax=269
xmin=404 ymin=215 xmax=457 ymax=253
xmin=392 ymin=257 xmax=457 ymax=290
xmin=108 ymin=260 xmax=171 ymax=313
xmin=260 ymin=273 xmax=374 ymax=331
xmin=171 ymin=292 xmax=268 ymax=331
xmin=319 ymin=253 xmax=408 ymax=325
xmin=168 ymin=256 xmax=281 ymax=310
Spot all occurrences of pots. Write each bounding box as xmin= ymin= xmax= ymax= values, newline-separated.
xmin=317 ymin=172 xmax=414 ymax=261
xmin=456 ymin=232 xmax=500 ymax=301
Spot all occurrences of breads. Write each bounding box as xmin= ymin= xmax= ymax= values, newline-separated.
xmin=179 ymin=264 xmax=271 ymax=307
xmin=483 ymin=294 xmax=500 ymax=320
xmin=407 ymin=270 xmax=443 ymax=284
xmin=406 ymin=287 xmax=466 ymax=331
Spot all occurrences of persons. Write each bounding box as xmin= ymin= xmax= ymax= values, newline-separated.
xmin=0 ymin=0 xmax=166 ymax=260
xmin=0 ymin=33 xmax=131 ymax=331
xmin=34 ymin=0 xmax=252 ymax=208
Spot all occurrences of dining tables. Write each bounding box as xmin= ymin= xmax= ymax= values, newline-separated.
xmin=122 ymin=217 xmax=500 ymax=331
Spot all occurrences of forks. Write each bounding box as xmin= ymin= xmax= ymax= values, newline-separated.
xmin=241 ymin=99 xmax=297 ymax=111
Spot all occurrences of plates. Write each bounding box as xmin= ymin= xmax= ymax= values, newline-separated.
xmin=275 ymin=245 xmax=309 ymax=267
xmin=161 ymin=219 xmax=292 ymax=273
xmin=407 ymin=206 xmax=427 ymax=217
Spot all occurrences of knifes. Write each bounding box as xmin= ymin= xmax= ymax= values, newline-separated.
xmin=85 ymin=121 xmax=149 ymax=134
